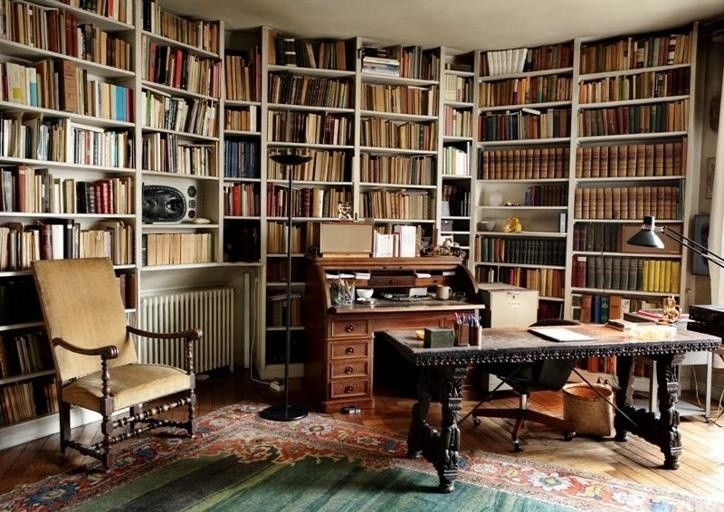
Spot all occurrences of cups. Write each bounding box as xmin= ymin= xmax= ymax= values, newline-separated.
xmin=437 ymin=284 xmax=453 ymax=301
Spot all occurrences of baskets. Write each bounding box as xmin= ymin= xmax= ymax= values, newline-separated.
xmin=562 ymin=383 xmax=614 ymax=436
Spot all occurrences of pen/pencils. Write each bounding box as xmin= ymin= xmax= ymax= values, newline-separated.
xmin=454 ymin=312 xmax=482 ymax=327
xmin=331 ymin=278 xmax=355 ymax=305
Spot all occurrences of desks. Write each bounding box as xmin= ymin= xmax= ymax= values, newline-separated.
xmin=373 ymin=317 xmax=721 ymax=488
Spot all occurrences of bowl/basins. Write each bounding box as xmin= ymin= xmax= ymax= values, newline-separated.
xmin=356 ymin=288 xmax=374 ymax=299
xmin=480 ymin=221 xmax=496 ymax=231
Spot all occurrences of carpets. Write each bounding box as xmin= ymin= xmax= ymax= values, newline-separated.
xmin=1 ymin=393 xmax=723 ymax=512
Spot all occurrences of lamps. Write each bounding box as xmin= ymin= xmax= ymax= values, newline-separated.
xmin=624 ymin=215 xmax=724 ymax=272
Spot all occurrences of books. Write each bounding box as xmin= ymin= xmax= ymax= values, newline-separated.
xmin=0 ymin=0 xmax=137 ymax=427
xmin=267 ymin=293 xmax=303 ymax=326
xmin=142 ymin=0 xmax=221 ymax=175
xmin=223 ymin=46 xmax=260 ymax=217
xmin=360 ymin=44 xmax=436 ymax=234
xmin=142 ymin=232 xmax=214 ymax=266
xmin=440 ymin=73 xmax=472 ymax=217
xmin=265 ymin=28 xmax=353 ymax=255
xmin=478 ymin=43 xmax=573 ymax=299
xmin=571 ymin=32 xmax=691 ymax=329
xmin=579 ymin=354 xmax=653 ymax=378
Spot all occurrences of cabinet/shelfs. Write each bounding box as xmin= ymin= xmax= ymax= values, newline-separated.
xmin=266 ymin=24 xmax=695 ymax=399
xmin=1 ymin=1 xmax=265 ymax=453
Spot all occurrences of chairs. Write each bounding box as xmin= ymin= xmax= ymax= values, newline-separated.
xmin=32 ymin=258 xmax=204 ymax=472
